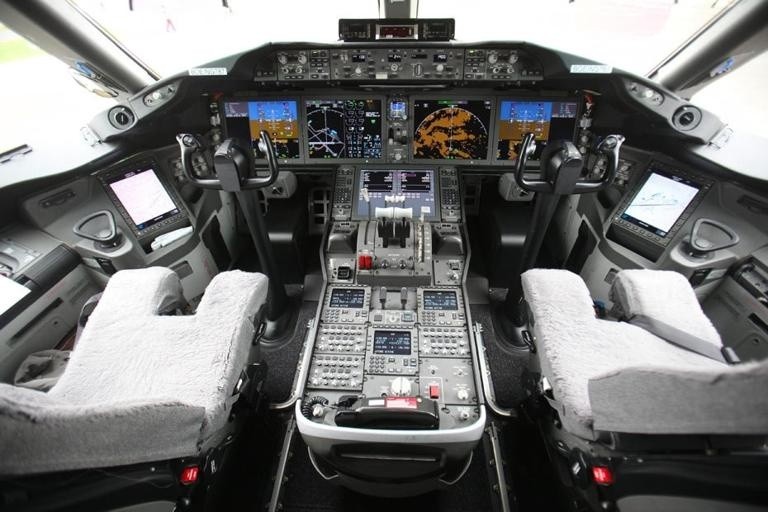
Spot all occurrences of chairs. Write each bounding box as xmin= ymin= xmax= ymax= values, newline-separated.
xmin=516 ymin=266 xmax=768 ymax=435
xmin=0 ymin=265 xmax=271 ymax=478
